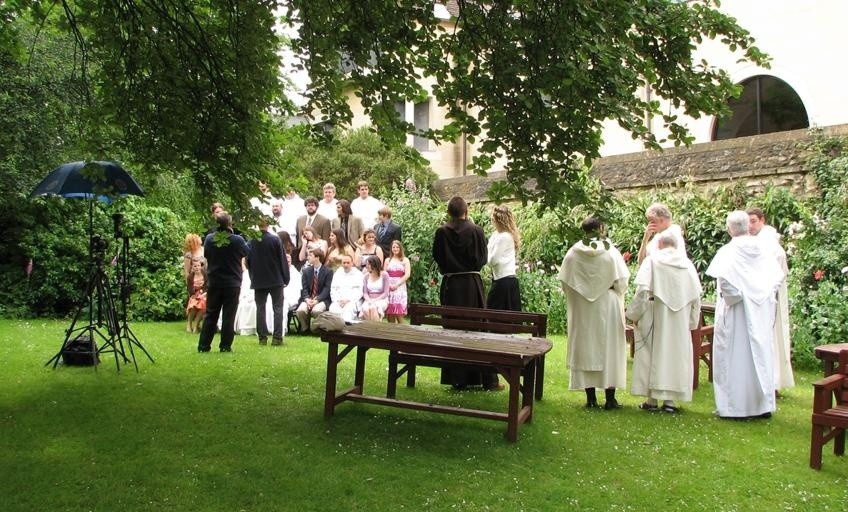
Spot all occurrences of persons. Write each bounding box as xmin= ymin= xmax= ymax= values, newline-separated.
xmin=183 ymin=180 xmax=411 ymax=352
xmin=626 ymin=234 xmax=703 ymax=412
xmin=432 ymin=196 xmax=505 ymax=391
xmin=558 ymin=217 xmax=631 ymax=409
xmin=486 ymin=205 xmax=522 ymax=334
xmin=639 ymin=203 xmax=687 ymax=265
xmin=705 ymin=210 xmax=785 ymax=418
xmin=747 ymin=207 xmax=794 ymax=399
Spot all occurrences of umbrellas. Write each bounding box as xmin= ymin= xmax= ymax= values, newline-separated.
xmin=26 ymin=160 xmax=145 ymax=341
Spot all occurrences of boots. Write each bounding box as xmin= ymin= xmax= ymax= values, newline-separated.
xmin=605 ymin=388 xmax=623 ymax=409
xmin=585 ymin=387 xmax=603 ymax=409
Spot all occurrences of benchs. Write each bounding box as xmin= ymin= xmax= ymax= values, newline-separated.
xmin=386 ymin=303 xmax=547 ymax=404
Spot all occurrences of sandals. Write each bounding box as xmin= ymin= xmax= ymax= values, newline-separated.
xmin=662 ymin=404 xmax=679 ymax=412
xmin=638 ymin=402 xmax=658 ymax=411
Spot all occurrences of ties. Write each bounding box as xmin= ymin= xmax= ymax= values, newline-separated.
xmin=310 ymin=271 xmax=318 ymax=299
xmin=308 ymin=217 xmax=312 ymax=224
xmin=341 ymin=217 xmax=346 ymax=237
xmin=378 ymin=223 xmax=384 ymax=242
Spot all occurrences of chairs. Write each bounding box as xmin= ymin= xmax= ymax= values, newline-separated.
xmin=635 ymin=303 xmax=713 ymax=389
xmin=810 ymin=349 xmax=848 ymax=469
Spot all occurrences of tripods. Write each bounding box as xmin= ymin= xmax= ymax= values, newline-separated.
xmin=49 ymin=205 xmax=121 ymax=372
xmin=97 ymin=240 xmax=155 ymax=377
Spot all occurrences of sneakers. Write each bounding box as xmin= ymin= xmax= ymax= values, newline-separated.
xmin=486 ymin=384 xmax=505 ymax=390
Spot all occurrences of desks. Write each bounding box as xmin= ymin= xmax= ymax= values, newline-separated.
xmin=815 ymin=342 xmax=848 ymax=432
xmin=324 ymin=320 xmax=551 ymax=442
